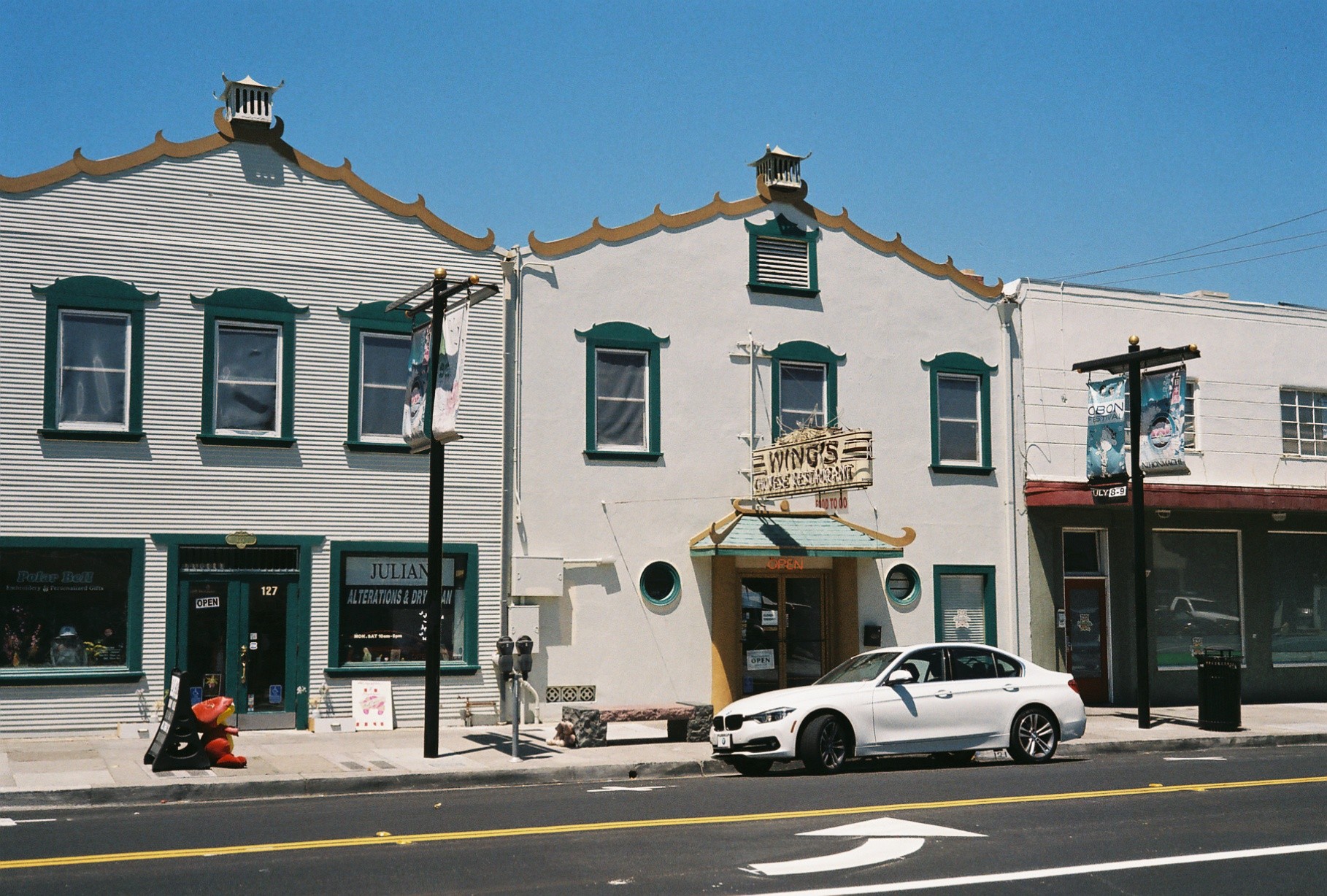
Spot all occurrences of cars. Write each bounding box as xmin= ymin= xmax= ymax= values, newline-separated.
xmin=708 ymin=642 xmax=1089 ymax=776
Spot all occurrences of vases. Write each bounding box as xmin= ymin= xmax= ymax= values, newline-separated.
xmin=312 ymin=708 xmax=322 ymax=717
xmin=150 ymin=713 xmax=158 ymax=722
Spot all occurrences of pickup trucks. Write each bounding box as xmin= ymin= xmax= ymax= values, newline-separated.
xmin=1154 ymin=596 xmax=1241 ymax=640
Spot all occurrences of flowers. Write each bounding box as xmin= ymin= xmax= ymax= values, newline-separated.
xmin=297 ymin=683 xmax=328 ymax=708
xmin=136 ymin=688 xmax=169 ymax=714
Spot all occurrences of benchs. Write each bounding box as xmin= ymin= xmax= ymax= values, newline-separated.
xmin=562 ymin=702 xmax=714 ymax=748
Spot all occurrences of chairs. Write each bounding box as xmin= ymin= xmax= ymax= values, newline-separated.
xmin=931 ymin=656 xmax=942 ymax=677
xmin=988 ymin=664 xmax=1005 ymax=672
xmin=973 ymin=663 xmax=986 ymax=671
xmin=901 ymin=663 xmax=920 ymax=682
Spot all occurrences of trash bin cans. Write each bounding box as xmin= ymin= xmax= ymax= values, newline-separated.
xmin=1195 ymin=646 xmax=1244 ymax=732
